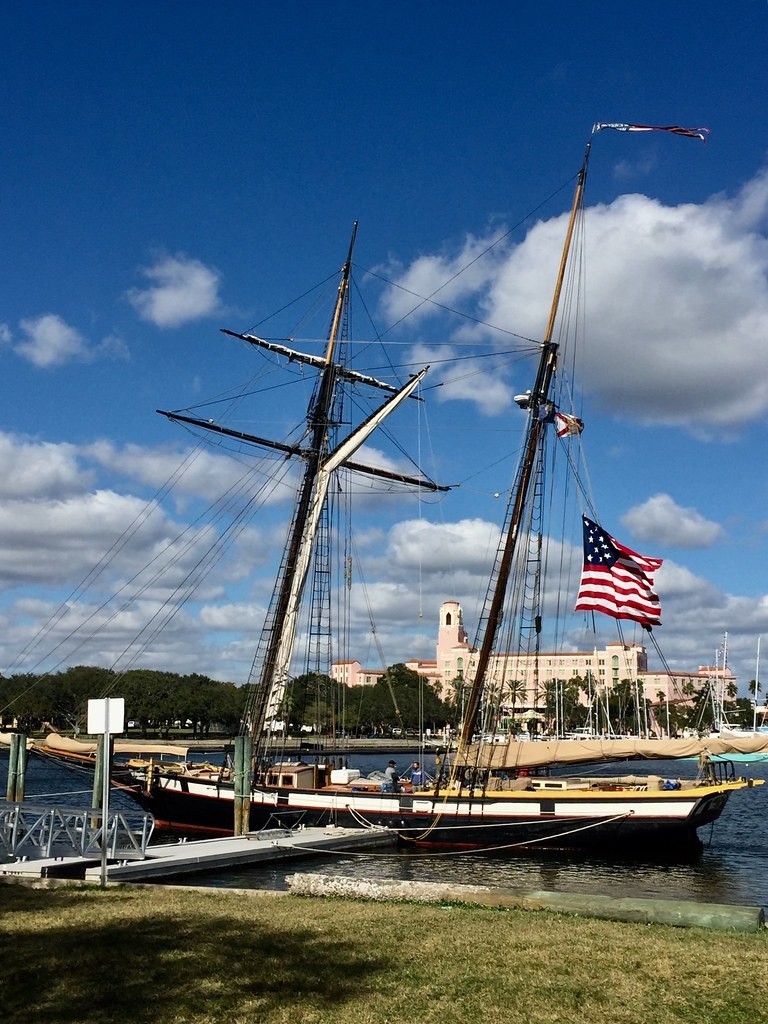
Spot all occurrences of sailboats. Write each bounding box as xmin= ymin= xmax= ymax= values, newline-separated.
xmin=30 ymin=122 xmax=766 ymax=866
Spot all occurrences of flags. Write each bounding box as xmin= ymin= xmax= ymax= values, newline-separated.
xmin=556 ymin=413 xmax=585 ymax=437
xmin=573 ymin=513 xmax=663 ymax=626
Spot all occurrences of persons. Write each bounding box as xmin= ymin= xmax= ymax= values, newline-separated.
xmin=385 ymin=760 xmax=400 ymax=793
xmin=410 ymin=762 xmax=426 ymax=792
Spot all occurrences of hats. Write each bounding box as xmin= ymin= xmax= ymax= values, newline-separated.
xmin=389 ymin=760 xmax=396 ymax=764
